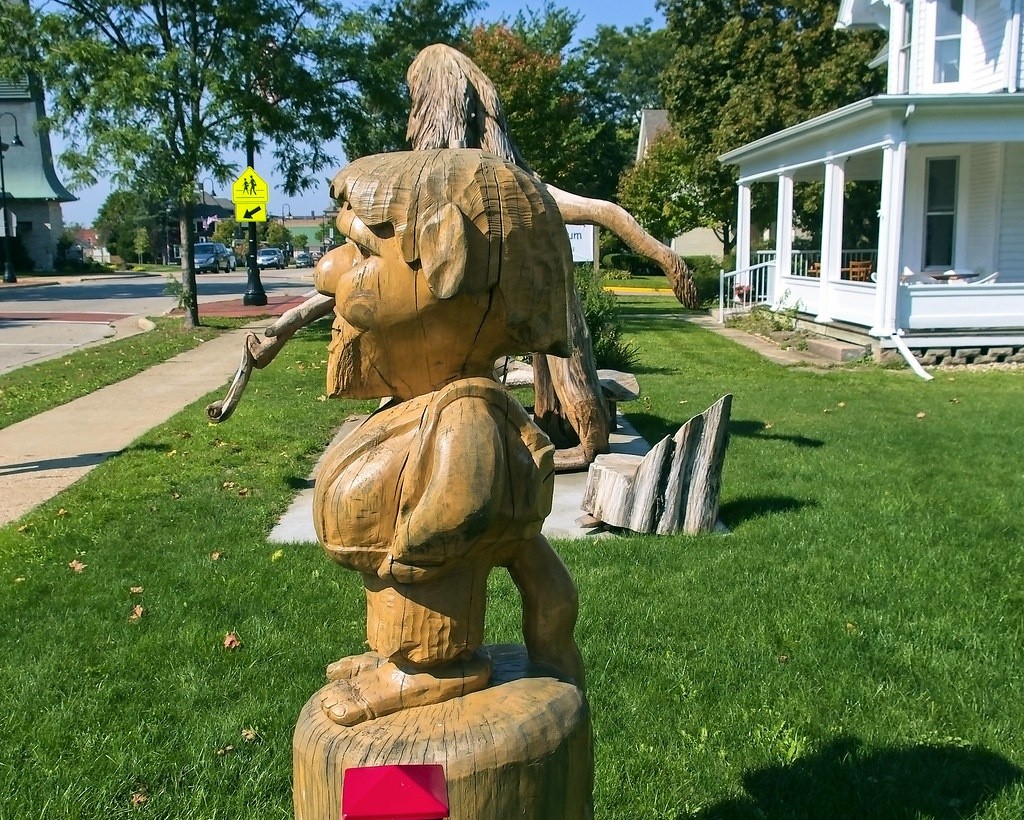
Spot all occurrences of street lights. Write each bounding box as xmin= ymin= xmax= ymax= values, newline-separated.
xmin=322 ymin=214 xmax=331 ymax=255
xmin=0 ymin=110 xmax=26 ymax=285
xmin=202 ymin=177 xmax=218 ymax=242
xmin=281 ymin=203 xmax=292 ymax=258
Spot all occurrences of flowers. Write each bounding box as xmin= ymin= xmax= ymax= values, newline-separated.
xmin=733 ymin=284 xmax=753 ymax=297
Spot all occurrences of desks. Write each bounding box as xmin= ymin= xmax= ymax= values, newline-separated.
xmin=808 ymin=268 xmax=870 ymax=271
xmin=899 ymin=271 xmax=979 ymax=281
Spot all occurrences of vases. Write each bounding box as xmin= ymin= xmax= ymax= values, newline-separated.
xmin=739 ymin=296 xmax=749 ymax=302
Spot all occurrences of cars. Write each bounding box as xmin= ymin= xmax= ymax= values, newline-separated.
xmin=193 ymin=243 xmax=232 ymax=273
xmin=226 ymin=247 xmax=237 ymax=270
xmin=295 ymin=253 xmax=315 ymax=267
xmin=257 ymin=248 xmax=286 ymax=270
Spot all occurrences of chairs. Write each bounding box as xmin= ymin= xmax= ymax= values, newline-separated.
xmin=815 ymin=263 xmax=820 ymax=277
xmin=968 ymin=271 xmax=1000 ymax=284
xmin=900 ymin=275 xmax=939 ymax=284
xmin=850 ymin=261 xmax=873 ymax=282
xmin=942 ymin=269 xmax=978 ymax=284
xmin=871 ymin=272 xmax=877 ymax=283
